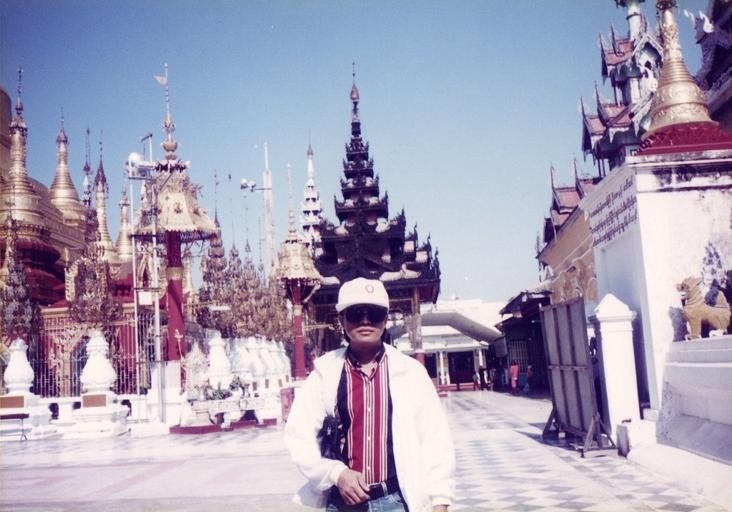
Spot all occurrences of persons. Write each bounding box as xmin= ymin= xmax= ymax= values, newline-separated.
xmin=283 ymin=275 xmax=457 ymax=512
xmin=470 ymin=360 xmax=536 ymax=397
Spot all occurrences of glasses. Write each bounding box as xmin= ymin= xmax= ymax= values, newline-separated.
xmin=343 ymin=306 xmax=388 ymax=324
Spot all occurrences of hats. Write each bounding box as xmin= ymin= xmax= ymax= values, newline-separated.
xmin=334 ymin=277 xmax=390 ymax=314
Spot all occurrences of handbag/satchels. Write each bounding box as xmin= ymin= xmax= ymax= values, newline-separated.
xmin=316 ymin=410 xmax=343 ymax=460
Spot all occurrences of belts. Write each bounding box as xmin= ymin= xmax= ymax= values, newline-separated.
xmin=330 ymin=476 xmax=399 ymax=503
